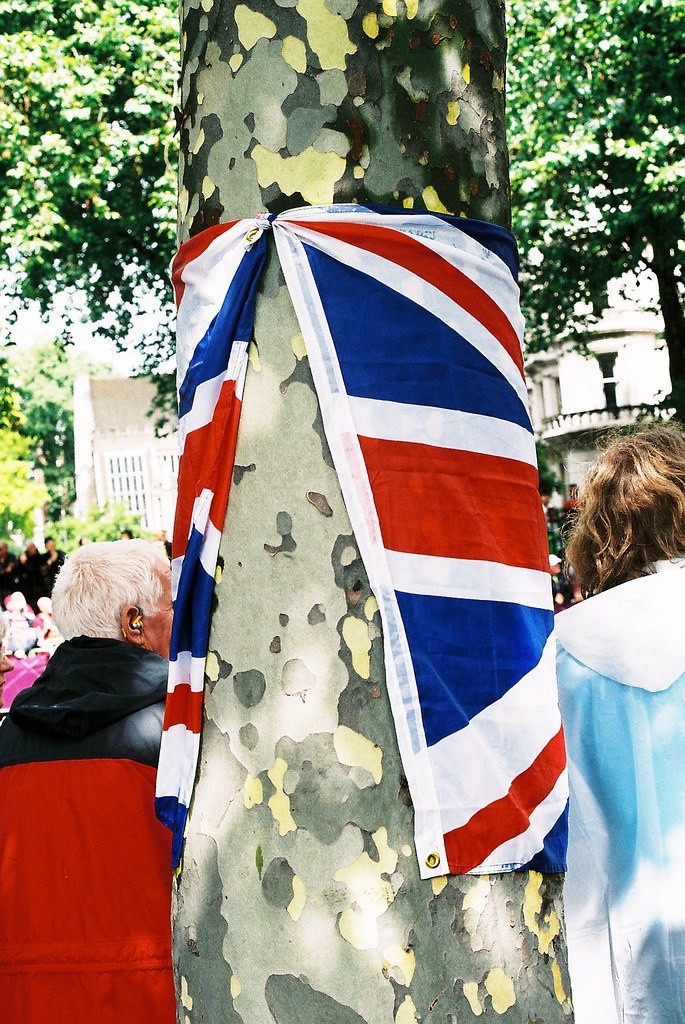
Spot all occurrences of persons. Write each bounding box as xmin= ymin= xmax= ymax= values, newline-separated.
xmin=0 ymin=481 xmax=592 ymax=1024
xmin=552 ymin=426 xmax=685 ymax=1022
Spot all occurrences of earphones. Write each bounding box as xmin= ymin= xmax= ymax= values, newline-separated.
xmin=130 ymin=615 xmax=142 ymax=632
